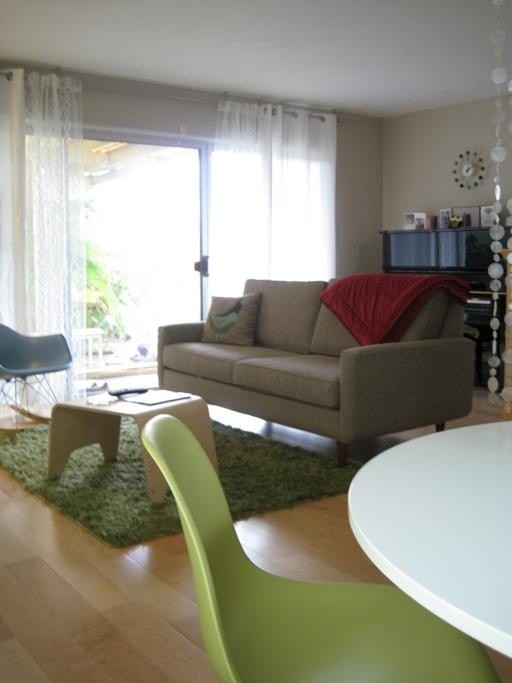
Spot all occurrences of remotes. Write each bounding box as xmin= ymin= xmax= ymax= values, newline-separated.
xmin=108 ymin=387 xmax=148 ymax=396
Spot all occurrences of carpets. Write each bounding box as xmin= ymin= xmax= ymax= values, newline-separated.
xmin=1 ymin=414 xmax=364 ymax=549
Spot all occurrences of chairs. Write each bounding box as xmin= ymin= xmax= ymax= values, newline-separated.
xmin=141 ymin=413 xmax=502 ymax=683
xmin=1 ymin=323 xmax=73 ymax=434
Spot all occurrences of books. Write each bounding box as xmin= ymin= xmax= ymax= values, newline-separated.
xmin=125 ymin=388 xmax=191 ymax=406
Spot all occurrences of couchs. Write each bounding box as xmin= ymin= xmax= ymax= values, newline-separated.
xmin=158 ymin=280 xmax=474 ymax=470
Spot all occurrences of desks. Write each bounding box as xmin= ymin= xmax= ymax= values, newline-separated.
xmin=345 ymin=419 xmax=512 ymax=660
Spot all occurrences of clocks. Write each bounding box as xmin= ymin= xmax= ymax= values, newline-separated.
xmin=453 ymin=150 xmax=485 ymax=190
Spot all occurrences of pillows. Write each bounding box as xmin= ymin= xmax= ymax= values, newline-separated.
xmin=244 ymin=279 xmax=328 ymax=356
xmin=309 ymin=281 xmax=448 ymax=355
xmin=201 ymin=292 xmax=262 ymax=347
